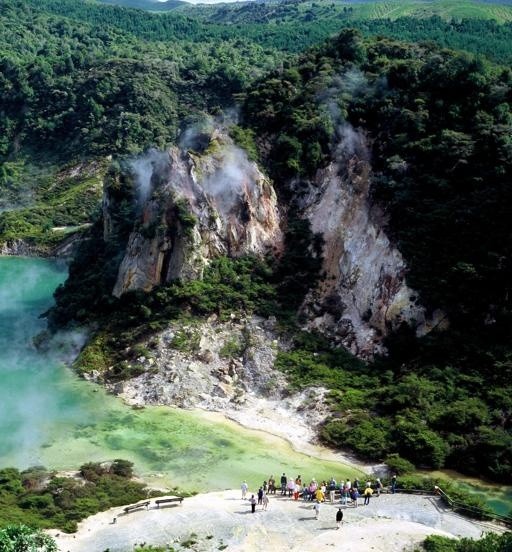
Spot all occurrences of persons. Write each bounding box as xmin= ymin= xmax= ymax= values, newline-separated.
xmin=241 ymin=479 xmax=248 ymax=499
xmin=249 ymin=472 xmax=397 ymax=528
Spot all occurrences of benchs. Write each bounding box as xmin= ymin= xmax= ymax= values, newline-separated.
xmin=124 ymin=497 xmax=184 ymax=513
